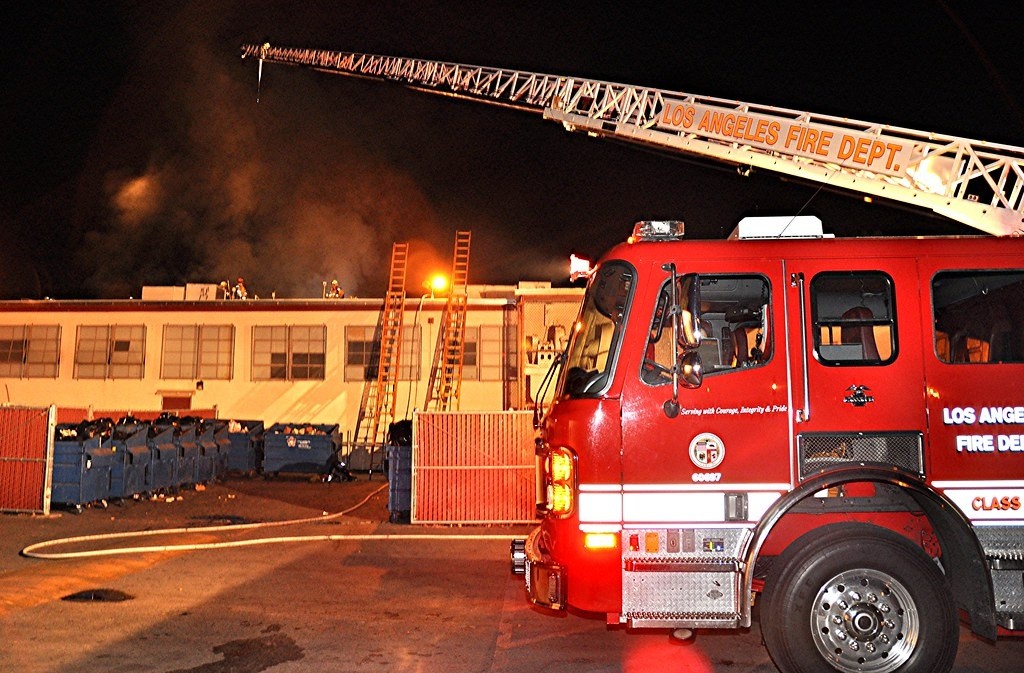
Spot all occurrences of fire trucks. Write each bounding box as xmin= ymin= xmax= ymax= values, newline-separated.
xmin=241 ymin=40 xmax=1024 ymax=672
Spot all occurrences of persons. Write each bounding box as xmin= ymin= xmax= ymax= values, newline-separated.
xmin=220 ymin=277 xmax=248 ymax=300
xmin=329 ymin=280 xmax=344 ymax=298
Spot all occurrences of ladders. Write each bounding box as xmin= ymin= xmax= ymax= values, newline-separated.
xmin=369 ymin=242 xmax=409 ymax=480
xmin=436 ymin=230 xmax=472 ymax=412
